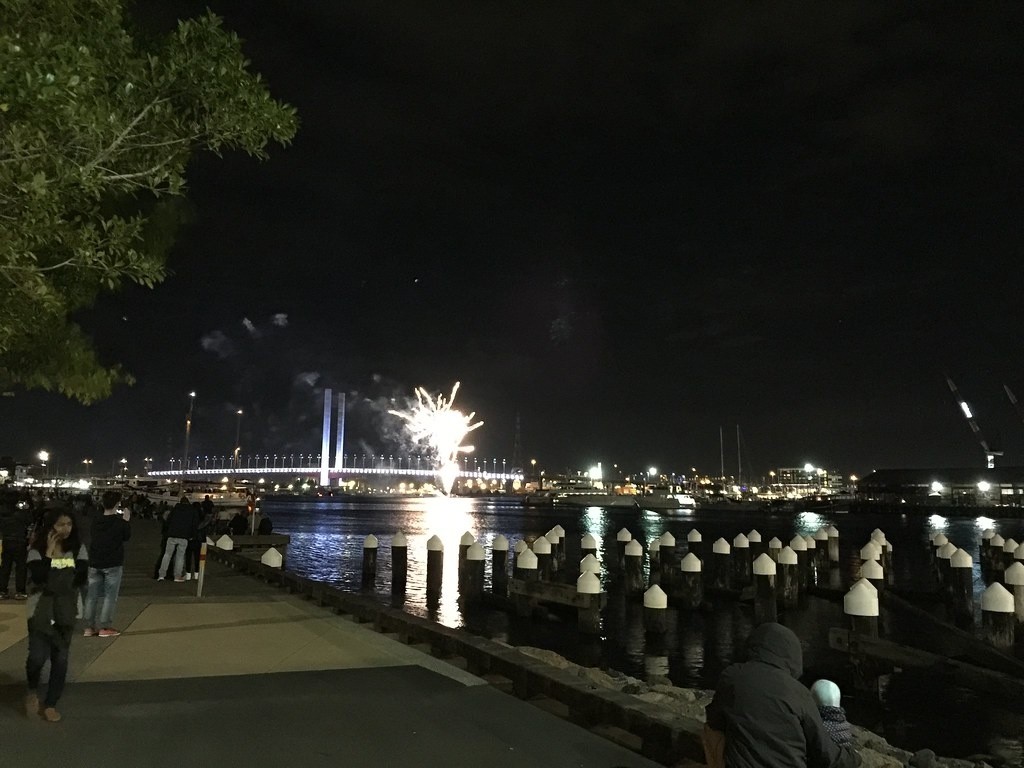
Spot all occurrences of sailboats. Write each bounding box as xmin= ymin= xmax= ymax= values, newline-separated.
xmin=704 ymin=424 xmax=770 ymax=511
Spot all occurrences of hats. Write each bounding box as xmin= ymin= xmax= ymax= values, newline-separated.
xmin=102 ymin=490 xmax=122 ymax=506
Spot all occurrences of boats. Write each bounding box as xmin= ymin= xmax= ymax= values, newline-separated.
xmin=522 ymin=481 xmax=700 ymax=510
xmin=91 ymin=475 xmax=257 ymax=526
xmin=766 ymin=492 xmax=839 ymax=514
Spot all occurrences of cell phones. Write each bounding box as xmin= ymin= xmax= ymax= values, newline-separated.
xmin=47 ymin=527 xmax=60 ymax=543
xmin=116 ymin=508 xmax=125 ymax=515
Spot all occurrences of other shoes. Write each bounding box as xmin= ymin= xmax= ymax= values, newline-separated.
xmin=45 ymin=707 xmax=62 ymax=722
xmin=14 ymin=593 xmax=28 ymax=600
xmin=174 ymin=576 xmax=185 ymax=584
xmin=0 ymin=591 xmax=11 ymax=600
xmin=99 ymin=627 xmax=121 ymax=637
xmin=192 ymin=572 xmax=199 ymax=580
xmin=24 ymin=693 xmax=39 ymax=715
xmin=83 ymin=627 xmax=99 ymax=637
xmin=158 ymin=577 xmax=165 ymax=583
xmin=182 ymin=572 xmax=193 ymax=580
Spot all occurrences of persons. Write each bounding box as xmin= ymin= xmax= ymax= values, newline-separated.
xmin=0 ymin=487 xmax=274 ymax=601
xmin=25 ymin=507 xmax=88 ymax=722
xmin=809 ymin=678 xmax=852 ymax=749
xmin=84 ymin=492 xmax=130 ymax=638
xmin=706 ymin=623 xmax=862 ymax=768
